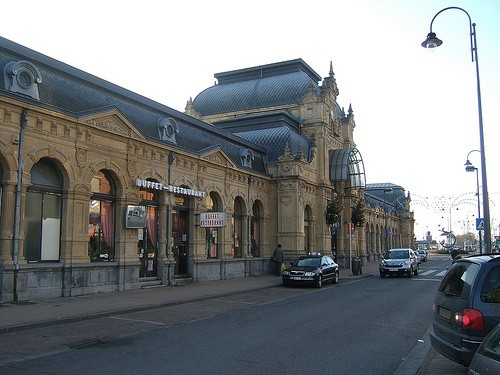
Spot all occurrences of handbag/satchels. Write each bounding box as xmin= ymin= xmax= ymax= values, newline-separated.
xmin=281 ymin=263 xmax=286 ymax=271
xmin=270 ymin=257 xmax=278 ymax=264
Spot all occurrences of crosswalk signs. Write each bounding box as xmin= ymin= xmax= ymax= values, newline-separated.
xmin=476 ymin=218 xmax=484 ymax=230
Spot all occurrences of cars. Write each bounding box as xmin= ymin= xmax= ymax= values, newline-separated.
xmin=281 ymin=252 xmax=340 ymax=288
xmin=413 ymin=251 xmax=421 ymax=265
xmin=465 ymin=321 xmax=500 ymax=375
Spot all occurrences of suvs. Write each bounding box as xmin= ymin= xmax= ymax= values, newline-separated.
xmin=416 ymin=250 xmax=427 ymax=262
xmin=429 ymin=252 xmax=500 ymax=368
xmin=378 ymin=248 xmax=419 ymax=277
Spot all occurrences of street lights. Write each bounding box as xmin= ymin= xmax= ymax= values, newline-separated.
xmin=465 ymin=166 xmax=482 ymax=254
xmin=420 ymin=5 xmax=490 ymax=252
xmin=464 ymin=149 xmax=491 ymax=250
xmin=442 ymin=207 xmax=459 ymax=249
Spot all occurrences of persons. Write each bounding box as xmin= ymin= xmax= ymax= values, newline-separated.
xmin=452 ymin=255 xmax=462 ymax=264
xmin=251 ymin=236 xmax=256 ymax=256
xmin=273 ymin=244 xmax=284 ymax=277
